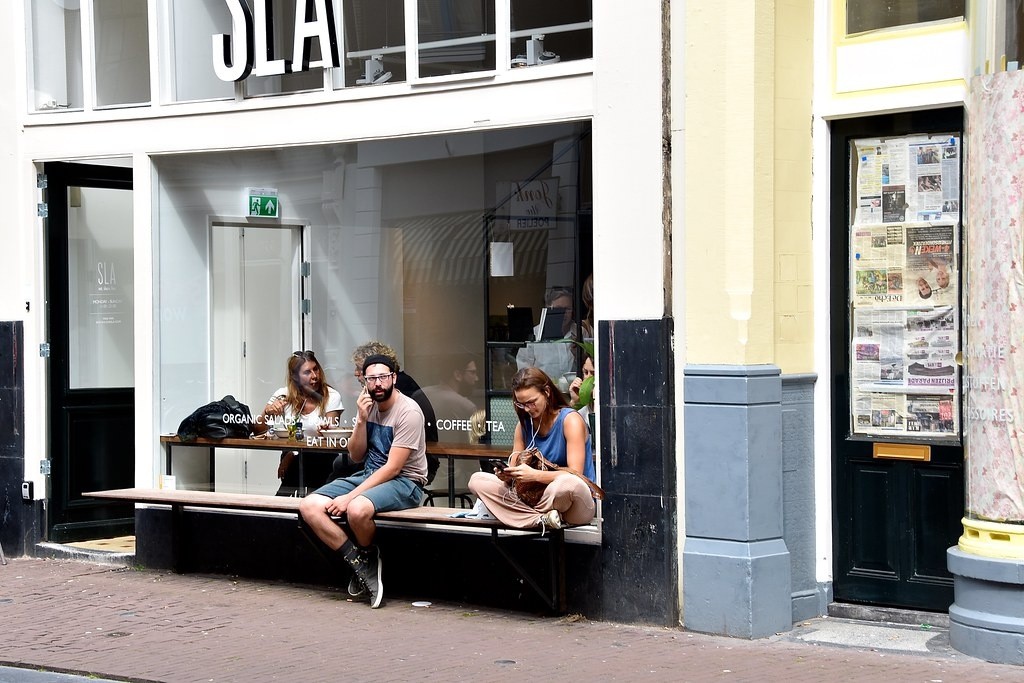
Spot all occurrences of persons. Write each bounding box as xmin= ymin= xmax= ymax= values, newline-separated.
xmin=532 ymin=285 xmax=594 ymax=343
xmin=907 ymin=253 xmax=951 ymax=300
xmin=468 ymin=367 xmax=595 ymax=528
xmin=411 ymin=346 xmax=482 ymax=509
xmin=352 ymin=340 xmax=440 ymax=487
xmin=298 ymin=354 xmax=428 ymax=609
xmin=253 ymin=350 xmax=345 ymax=497
xmin=566 ymin=356 xmax=595 ymax=430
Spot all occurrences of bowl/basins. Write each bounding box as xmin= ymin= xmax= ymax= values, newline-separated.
xmin=272 ymin=428 xmax=290 ymax=438
xmin=320 ymin=430 xmax=353 ymax=440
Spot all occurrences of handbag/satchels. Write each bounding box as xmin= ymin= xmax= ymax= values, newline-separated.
xmin=178 ymin=394 xmax=254 ymax=444
xmin=515 ymin=446 xmax=603 ymax=507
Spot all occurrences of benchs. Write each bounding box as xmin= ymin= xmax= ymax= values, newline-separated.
xmin=78 ymin=482 xmax=565 ymax=621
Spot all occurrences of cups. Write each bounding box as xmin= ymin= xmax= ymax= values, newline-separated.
xmin=286 ymin=417 xmax=301 ymax=440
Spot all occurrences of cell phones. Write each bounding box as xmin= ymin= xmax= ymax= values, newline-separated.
xmin=364 ymin=381 xmax=369 ymax=394
xmin=488 ymin=459 xmax=508 ymax=472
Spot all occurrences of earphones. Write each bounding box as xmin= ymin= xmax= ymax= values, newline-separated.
xmin=545 ymin=391 xmax=547 ymax=394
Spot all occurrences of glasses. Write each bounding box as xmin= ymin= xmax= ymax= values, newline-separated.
xmin=514 ymin=394 xmax=542 ymax=409
xmin=363 ymin=372 xmax=393 ymax=384
xmin=292 ymin=350 xmax=314 ymax=358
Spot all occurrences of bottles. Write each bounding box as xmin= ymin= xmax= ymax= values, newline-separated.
xmin=296 ymin=422 xmax=304 ymax=443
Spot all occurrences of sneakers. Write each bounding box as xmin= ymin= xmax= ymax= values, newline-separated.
xmin=354 ymin=545 xmax=384 ymax=609
xmin=542 ymin=509 xmax=561 ymax=529
xmin=348 ymin=572 xmax=367 ymax=597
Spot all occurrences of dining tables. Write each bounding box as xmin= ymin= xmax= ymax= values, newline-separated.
xmin=160 ymin=416 xmax=517 ymax=512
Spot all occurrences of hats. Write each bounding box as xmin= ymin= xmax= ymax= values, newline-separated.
xmin=363 ymin=354 xmax=395 ymax=380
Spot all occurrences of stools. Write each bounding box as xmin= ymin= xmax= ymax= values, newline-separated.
xmin=423 ymin=484 xmax=476 ymax=509
